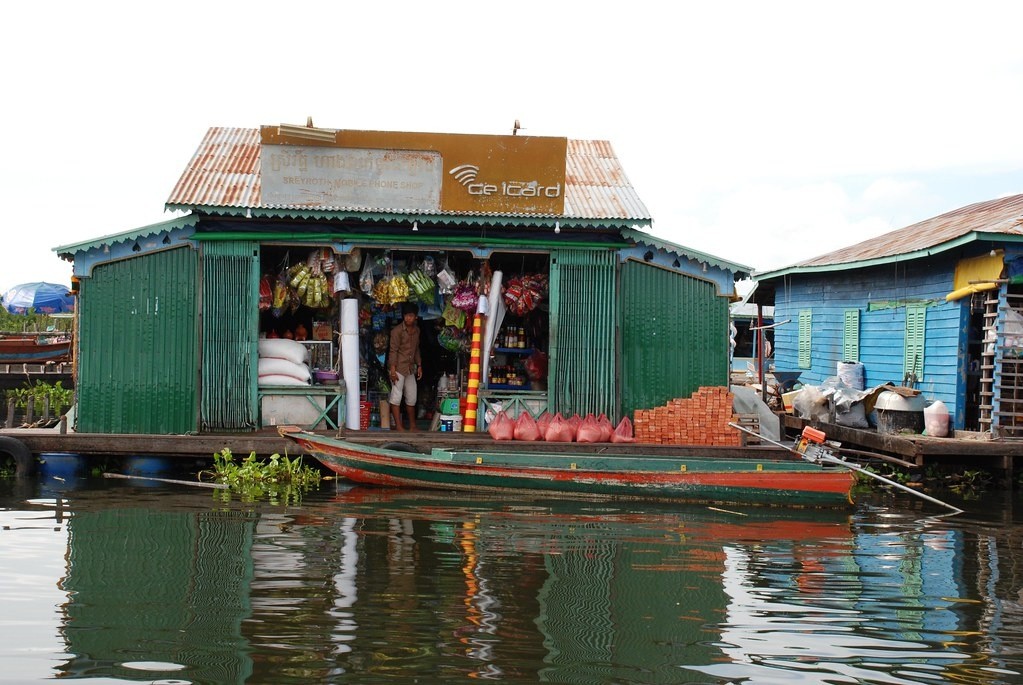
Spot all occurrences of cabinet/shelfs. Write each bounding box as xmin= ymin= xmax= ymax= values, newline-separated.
xmin=488 ymin=348 xmax=535 ymax=389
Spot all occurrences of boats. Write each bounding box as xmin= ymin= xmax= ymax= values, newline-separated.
xmin=277 ymin=424 xmax=860 ymax=512
xmin=0 ymin=330 xmax=74 ymax=364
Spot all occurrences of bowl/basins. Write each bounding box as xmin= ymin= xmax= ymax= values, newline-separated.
xmin=873 ymin=391 xmax=927 ymax=412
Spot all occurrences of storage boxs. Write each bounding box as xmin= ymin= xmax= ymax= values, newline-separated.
xmin=782 ymin=389 xmax=803 ymax=413
xmin=360 ymin=402 xmax=372 ymax=430
xmin=366 ymin=391 xmax=390 ymax=431
xmin=733 ymin=412 xmax=762 ymax=444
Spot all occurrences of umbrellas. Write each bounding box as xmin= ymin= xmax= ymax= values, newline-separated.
xmin=0 ymin=282 xmax=79 ymax=332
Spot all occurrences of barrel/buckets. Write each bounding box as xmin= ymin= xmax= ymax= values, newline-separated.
xmin=40 ymin=453 xmax=79 ymax=484
xmin=124 ymin=457 xmax=162 ymax=487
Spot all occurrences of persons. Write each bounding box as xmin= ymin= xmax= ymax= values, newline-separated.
xmin=388 ymin=303 xmax=425 ymax=433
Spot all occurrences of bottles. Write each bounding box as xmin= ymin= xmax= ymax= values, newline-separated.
xmin=439 ymin=372 xmax=459 ymax=391
xmin=491 ymin=327 xmax=530 ymax=385
xmin=370 ymin=407 xmax=380 ymax=428
xmin=270 ymin=324 xmax=307 ymax=342
